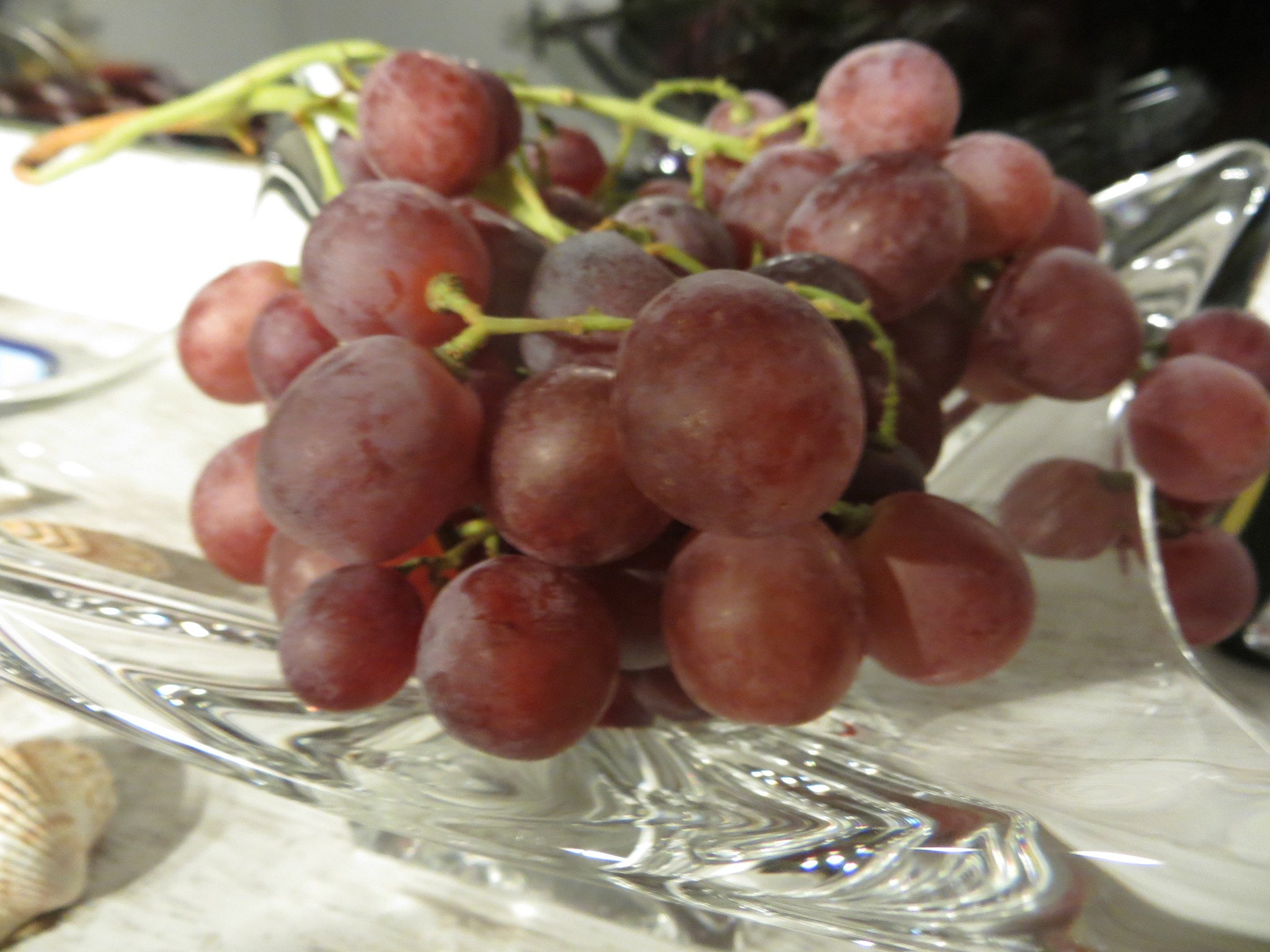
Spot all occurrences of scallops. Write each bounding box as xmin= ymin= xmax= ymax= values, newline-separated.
xmin=0 ymin=736 xmax=116 ymax=949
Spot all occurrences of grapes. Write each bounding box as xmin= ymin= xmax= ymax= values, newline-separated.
xmin=175 ymin=38 xmax=1270 ymax=761
